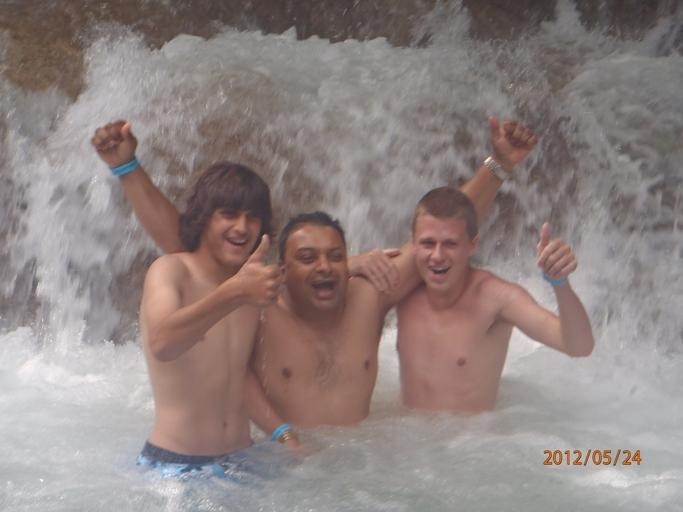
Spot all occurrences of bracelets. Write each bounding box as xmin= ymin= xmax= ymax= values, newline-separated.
xmin=541 ymin=273 xmax=568 ymax=286
xmin=109 ymin=157 xmax=140 ymax=178
xmin=278 ymin=430 xmax=298 ymax=444
xmin=483 ymin=155 xmax=509 ymax=183
xmin=270 ymin=423 xmax=293 ymax=443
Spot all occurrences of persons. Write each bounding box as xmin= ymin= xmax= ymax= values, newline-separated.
xmin=87 ymin=114 xmax=546 ymax=446
xmin=132 ymin=160 xmax=304 ymax=487
xmin=339 ymin=183 xmax=599 ymax=420
